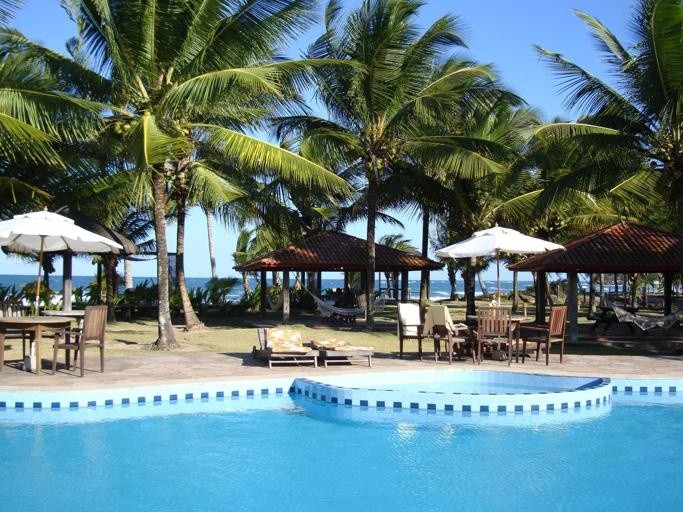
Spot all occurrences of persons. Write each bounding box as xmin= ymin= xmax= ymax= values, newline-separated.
xmin=331 ymin=287 xmax=344 ymax=308
xmin=342 ymin=286 xmax=355 ymax=308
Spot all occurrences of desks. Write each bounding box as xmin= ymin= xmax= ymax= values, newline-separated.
xmin=468 ymin=315 xmax=519 ymax=363
xmin=0 ymin=316 xmax=75 ymax=375
xmin=41 ymin=310 xmax=86 ymax=371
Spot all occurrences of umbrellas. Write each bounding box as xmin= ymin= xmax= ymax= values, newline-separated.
xmin=1 ymin=205 xmax=125 ymax=315
xmin=433 ymin=220 xmax=564 ymax=306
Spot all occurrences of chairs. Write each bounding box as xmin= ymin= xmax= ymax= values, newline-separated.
xmin=254 ymin=326 xmax=320 ymax=368
xmin=429 ymin=305 xmax=478 ymax=363
xmin=474 ymin=307 xmax=513 ymax=365
xmin=3 ymin=301 xmax=34 ymax=361
xmin=398 ymin=302 xmax=443 ymax=361
xmin=50 ymin=305 xmax=108 ymax=377
xmin=312 ymin=338 xmax=374 ymax=368
xmin=517 ymin=306 xmax=567 ymax=366
